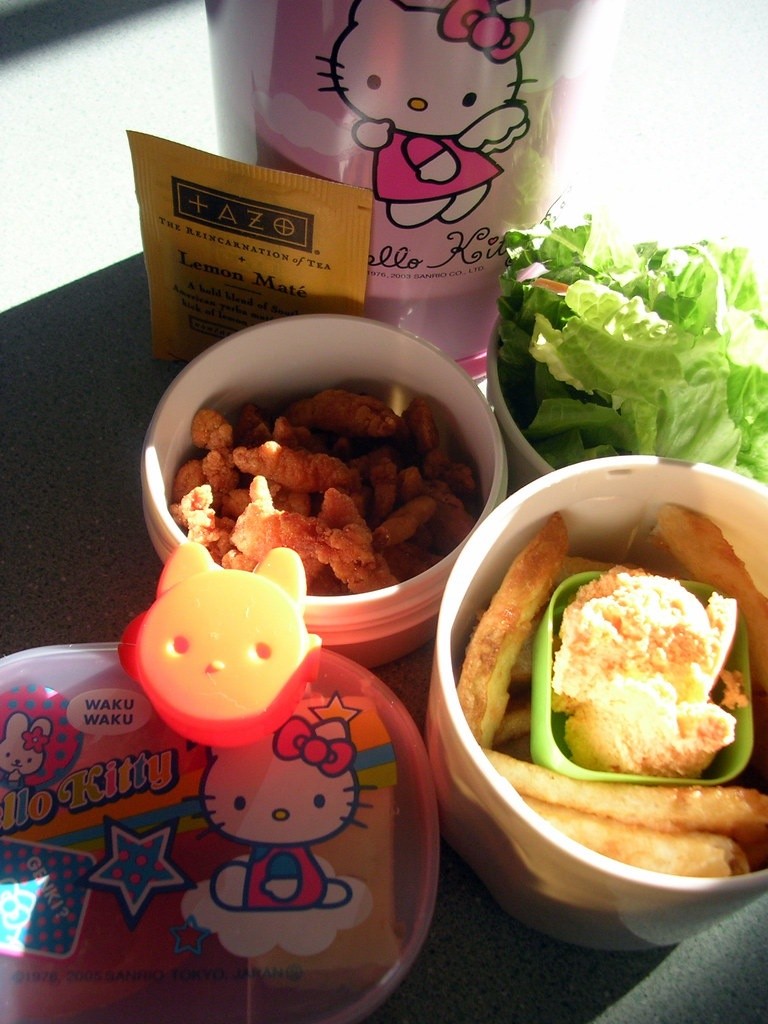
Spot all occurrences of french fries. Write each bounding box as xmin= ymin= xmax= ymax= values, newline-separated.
xmin=453 ymin=501 xmax=768 ymax=879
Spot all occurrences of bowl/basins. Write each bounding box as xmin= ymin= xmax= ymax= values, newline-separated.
xmin=531 ymin=572 xmax=754 ymax=786
xmin=424 ymin=454 xmax=768 ymax=952
xmin=483 ymin=313 xmax=594 ymax=492
xmin=142 ymin=314 xmax=507 ymax=670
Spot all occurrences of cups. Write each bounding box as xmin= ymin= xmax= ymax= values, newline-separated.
xmin=201 ymin=0 xmax=589 ymax=380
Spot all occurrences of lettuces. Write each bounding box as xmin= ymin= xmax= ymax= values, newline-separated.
xmin=494 ymin=208 xmax=768 ymax=489
